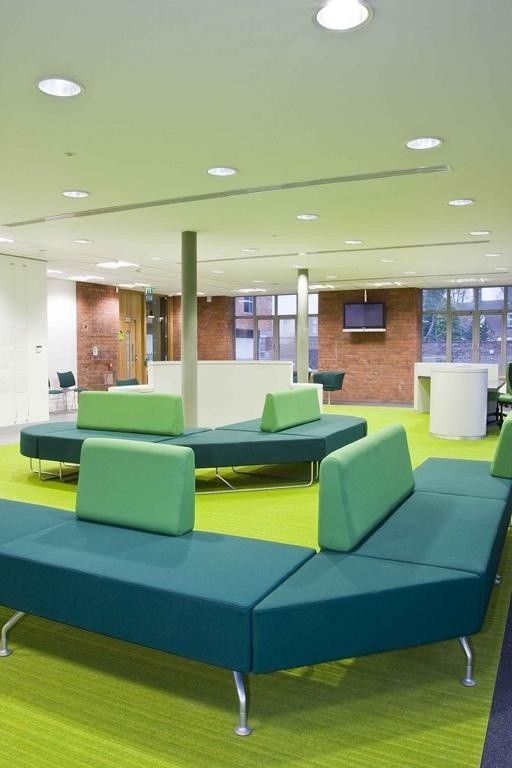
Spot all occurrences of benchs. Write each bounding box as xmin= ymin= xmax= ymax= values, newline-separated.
xmin=0 ymin=388 xmax=512 ymax=737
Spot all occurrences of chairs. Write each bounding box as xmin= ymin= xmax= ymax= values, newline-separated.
xmin=116 ymin=378 xmax=138 ymax=386
xmin=48 ymin=371 xmax=89 ymax=412
xmin=293 ymin=371 xmax=311 ymax=383
xmin=313 ymin=371 xmax=345 ymax=405
xmin=487 ymin=362 xmax=512 ymax=430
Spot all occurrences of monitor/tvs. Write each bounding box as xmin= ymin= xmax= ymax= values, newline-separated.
xmin=343 ymin=302 xmax=386 ymax=333
